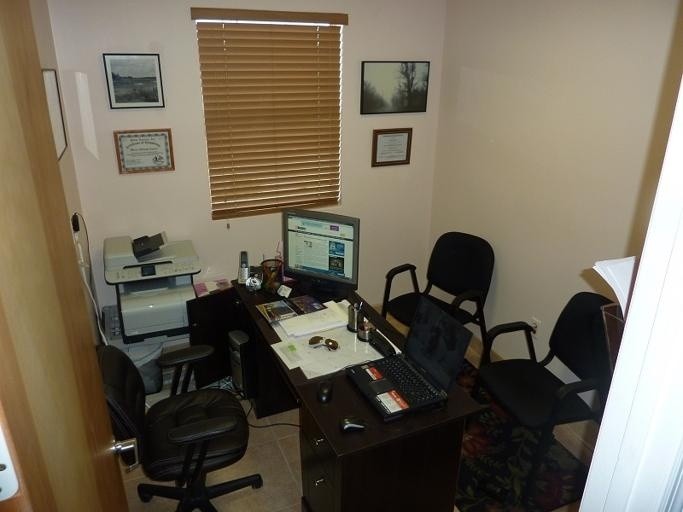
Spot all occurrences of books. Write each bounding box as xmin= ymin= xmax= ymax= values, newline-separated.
xmin=255 ymin=293 xmax=349 ymax=337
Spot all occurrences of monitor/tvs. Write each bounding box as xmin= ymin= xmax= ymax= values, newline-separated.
xmin=283 ymin=208 xmax=360 ymax=301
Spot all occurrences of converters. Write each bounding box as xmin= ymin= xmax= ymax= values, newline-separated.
xmin=73 ymin=215 xmax=79 ymax=231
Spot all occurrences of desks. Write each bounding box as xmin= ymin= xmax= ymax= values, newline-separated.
xmin=184 ymin=273 xmax=481 ymax=512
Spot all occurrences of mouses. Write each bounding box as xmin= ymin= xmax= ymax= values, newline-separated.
xmin=340 ymin=415 xmax=368 ymax=432
xmin=317 ymin=378 xmax=333 ymax=403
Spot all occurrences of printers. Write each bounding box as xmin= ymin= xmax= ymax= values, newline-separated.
xmin=103 ymin=231 xmax=201 ymax=345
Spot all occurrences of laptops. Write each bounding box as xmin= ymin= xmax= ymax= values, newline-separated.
xmin=345 ymin=295 xmax=473 ymax=419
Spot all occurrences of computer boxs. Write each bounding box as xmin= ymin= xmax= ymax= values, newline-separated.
xmin=227 ymin=325 xmax=258 ymax=400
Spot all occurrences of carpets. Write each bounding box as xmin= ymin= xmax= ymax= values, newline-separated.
xmin=451 ymin=357 xmax=588 ymax=512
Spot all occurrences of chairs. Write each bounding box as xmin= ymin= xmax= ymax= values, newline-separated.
xmin=380 ymin=230 xmax=495 ymax=348
xmin=469 ymin=292 xmax=626 ymax=506
xmin=94 ymin=344 xmax=261 ymax=511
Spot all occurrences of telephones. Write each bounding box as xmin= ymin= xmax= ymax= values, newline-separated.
xmin=238 ymin=250 xmax=250 ymax=284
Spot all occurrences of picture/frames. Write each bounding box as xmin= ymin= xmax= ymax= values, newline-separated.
xmin=101 ymin=52 xmax=164 ymax=110
xmin=358 ymin=61 xmax=430 ymax=115
